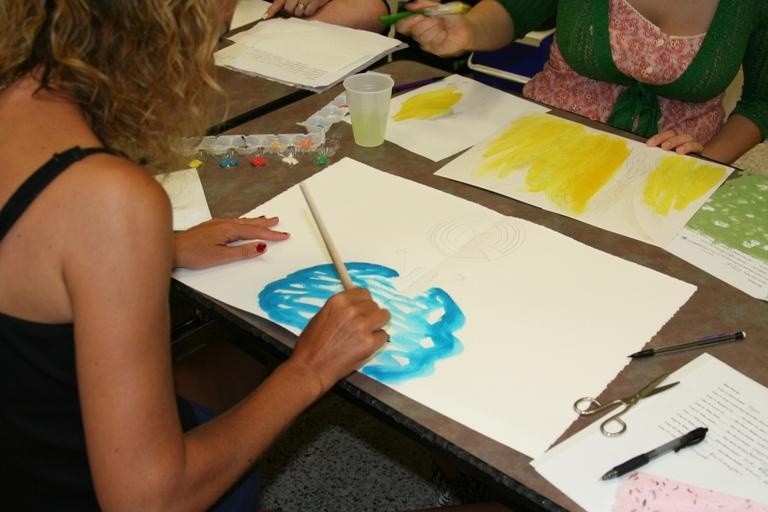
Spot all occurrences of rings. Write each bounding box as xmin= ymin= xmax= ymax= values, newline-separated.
xmin=295 ymin=2 xmax=310 ymax=10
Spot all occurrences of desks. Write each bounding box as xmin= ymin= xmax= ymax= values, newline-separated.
xmin=110 ymin=0 xmax=767 ymax=510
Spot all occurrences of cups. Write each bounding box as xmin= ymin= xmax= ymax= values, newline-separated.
xmin=342 ymin=73 xmax=394 ymax=148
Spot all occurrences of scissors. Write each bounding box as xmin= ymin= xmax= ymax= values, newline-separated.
xmin=573 ymin=371 xmax=681 ymax=437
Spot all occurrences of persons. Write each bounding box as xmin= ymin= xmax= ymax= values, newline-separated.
xmin=392 ymin=2 xmax=768 ymax=165
xmin=262 ymin=0 xmax=399 ymax=39
xmin=0 ymin=0 xmax=394 ymax=512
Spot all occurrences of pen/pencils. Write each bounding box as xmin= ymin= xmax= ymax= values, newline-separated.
xmin=392 ymin=76 xmax=447 ymax=96
xmin=599 ymin=427 xmax=709 ymax=483
xmin=300 ymin=183 xmax=353 ymax=292
xmin=379 ymin=2 xmax=472 ymax=25
xmin=626 ymin=331 xmax=747 ymax=363
